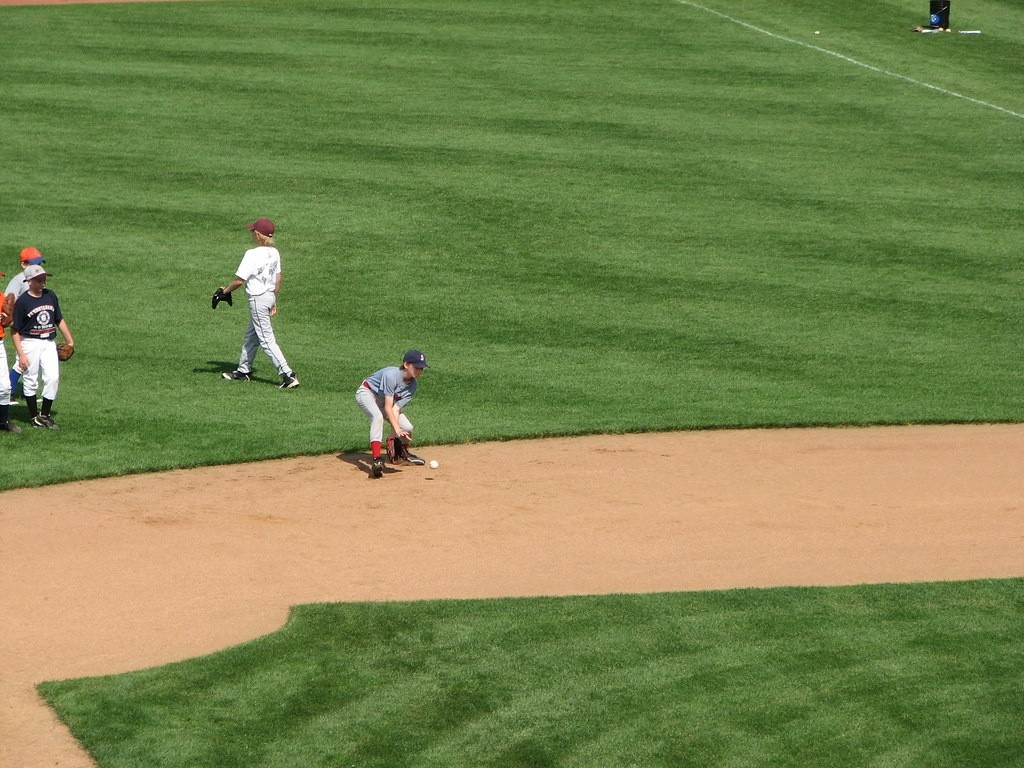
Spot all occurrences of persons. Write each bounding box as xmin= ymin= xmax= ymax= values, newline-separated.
xmin=355 ymin=350 xmax=431 ymax=478
xmin=0 ymin=247 xmax=76 ymax=434
xmin=220 ymin=217 xmax=299 ymax=388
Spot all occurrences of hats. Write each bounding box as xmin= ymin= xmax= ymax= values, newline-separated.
xmin=403 ymin=349 xmax=431 ymax=369
xmin=23 ymin=264 xmax=52 ymax=282
xmin=247 ymin=217 xmax=274 ymax=237
xmin=19 ymin=247 xmax=46 ymax=264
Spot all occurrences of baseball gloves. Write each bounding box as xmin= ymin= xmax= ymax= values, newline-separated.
xmin=2 ymin=293 xmax=15 ymax=328
xmin=387 ymin=433 xmax=408 ymax=465
xmin=212 ymin=288 xmax=232 ymax=309
xmin=56 ymin=343 xmax=74 ymax=361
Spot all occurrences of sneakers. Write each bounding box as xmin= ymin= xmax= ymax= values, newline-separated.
xmin=279 ymin=373 xmax=299 ymax=388
xmin=222 ymin=371 xmax=256 ymax=382
xmin=31 ymin=416 xmax=39 ymax=428
xmin=371 ymin=459 xmax=384 ymax=478
xmin=35 ymin=411 xmax=60 ymax=431
xmin=405 ymin=451 xmax=426 ymax=465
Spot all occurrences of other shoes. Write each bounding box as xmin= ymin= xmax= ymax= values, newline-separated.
xmin=36 ymin=397 xmax=43 ymax=403
xmin=0 ymin=421 xmax=22 ymax=433
xmin=10 ymin=394 xmax=19 ymax=404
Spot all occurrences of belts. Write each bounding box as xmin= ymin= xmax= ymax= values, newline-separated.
xmin=363 ymin=381 xmax=371 ymax=389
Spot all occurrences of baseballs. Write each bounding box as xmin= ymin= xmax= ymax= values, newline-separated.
xmin=430 ymin=460 xmax=438 ymax=469
